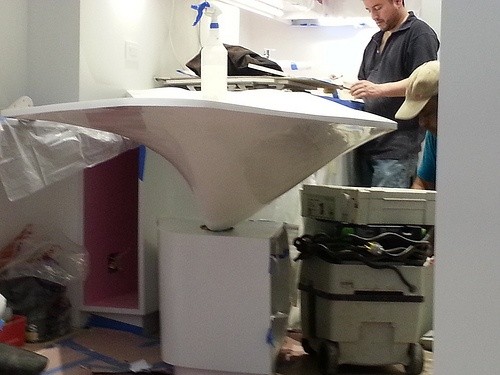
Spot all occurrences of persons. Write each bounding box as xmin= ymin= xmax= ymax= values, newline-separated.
xmin=394 ymin=60 xmax=440 ymax=191
xmin=349 ymin=0 xmax=441 ymax=188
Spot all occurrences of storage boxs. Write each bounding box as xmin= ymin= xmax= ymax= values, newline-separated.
xmin=300 ymin=183 xmax=436 ymax=225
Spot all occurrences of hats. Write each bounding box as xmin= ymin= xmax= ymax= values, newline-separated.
xmin=394 ymin=60 xmax=439 ymax=120
